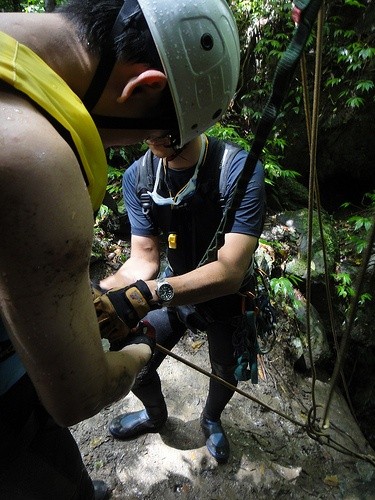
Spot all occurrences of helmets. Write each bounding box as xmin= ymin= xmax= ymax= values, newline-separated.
xmin=137 ymin=0 xmax=240 ymax=149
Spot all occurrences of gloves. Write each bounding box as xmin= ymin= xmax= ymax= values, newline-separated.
xmin=92 ymin=280 xmax=158 ymax=344
xmin=132 ymin=319 xmax=156 ymax=355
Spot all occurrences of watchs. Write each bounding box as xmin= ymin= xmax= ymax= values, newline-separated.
xmin=153 ymin=278 xmax=175 ymax=307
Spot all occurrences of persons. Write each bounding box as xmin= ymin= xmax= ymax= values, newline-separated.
xmin=87 ymin=129 xmax=267 ymax=462
xmin=0 ymin=0 xmax=242 ymax=499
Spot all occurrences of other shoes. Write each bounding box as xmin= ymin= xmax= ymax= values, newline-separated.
xmin=108 ymin=410 xmax=164 ymax=438
xmin=200 ymin=412 xmax=228 ymax=458
xmin=92 ymin=480 xmax=110 ymax=500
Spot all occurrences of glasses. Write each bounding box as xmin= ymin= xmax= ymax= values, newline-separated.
xmin=147 ymin=133 xmax=206 ymax=205
